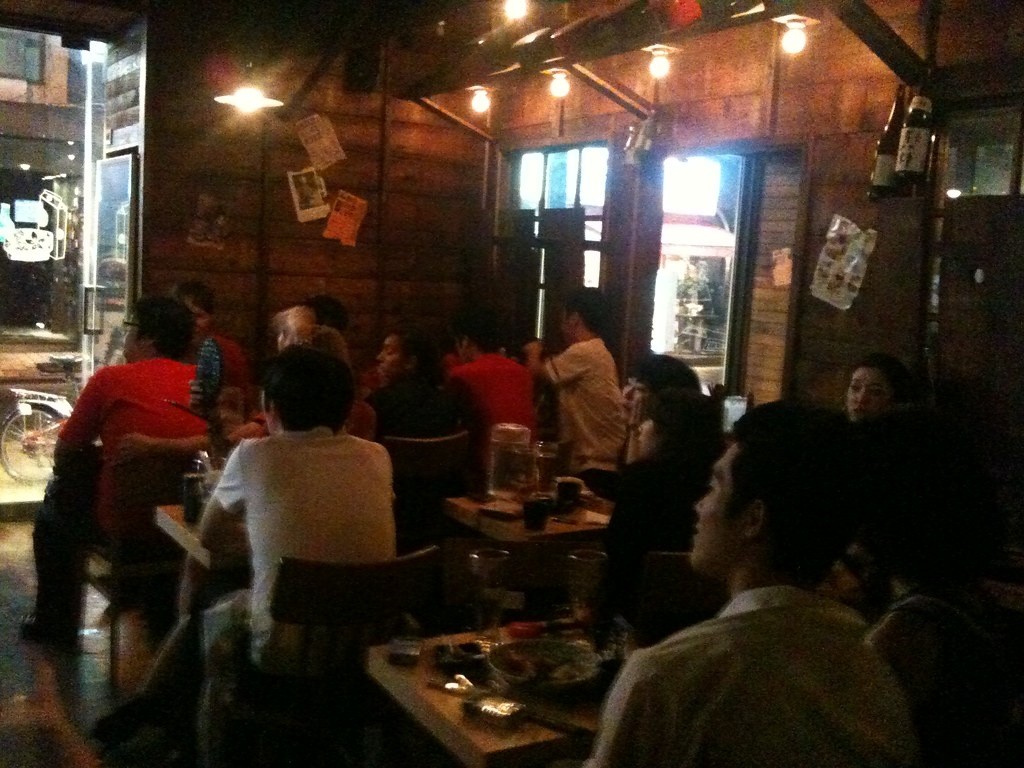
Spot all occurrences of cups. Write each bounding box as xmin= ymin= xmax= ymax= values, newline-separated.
xmin=557 ymin=482 xmax=580 ymax=508
xmin=566 ymin=548 xmax=607 ymax=637
xmin=485 ymin=423 xmax=539 ymax=504
xmin=524 ymin=501 xmax=548 ymax=529
xmin=468 ymin=548 xmax=510 ymax=649
xmin=198 ymin=458 xmax=226 ymax=502
xmin=184 ymin=472 xmax=206 ymax=521
xmin=534 ymin=440 xmax=558 ymax=497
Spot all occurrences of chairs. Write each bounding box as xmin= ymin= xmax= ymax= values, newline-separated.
xmin=84 ymin=428 xmax=713 ymax=768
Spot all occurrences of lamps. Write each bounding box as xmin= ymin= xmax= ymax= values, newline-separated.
xmin=214 ymin=59 xmax=283 ymax=113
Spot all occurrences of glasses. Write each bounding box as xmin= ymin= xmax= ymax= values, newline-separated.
xmin=121 ymin=318 xmax=143 ymax=330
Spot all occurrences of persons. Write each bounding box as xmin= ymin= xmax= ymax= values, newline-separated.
xmin=19 ymin=297 xmax=206 ymax=640
xmin=19 ymin=275 xmax=1024 ymax=768
xmin=585 ymin=399 xmax=915 ymax=768
xmin=92 ymin=345 xmax=393 ymax=767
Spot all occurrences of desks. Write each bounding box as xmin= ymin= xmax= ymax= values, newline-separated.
xmin=445 ymin=495 xmax=612 ymax=540
xmin=367 ymin=618 xmax=635 ymax=767
xmin=153 ymin=503 xmax=247 ymax=573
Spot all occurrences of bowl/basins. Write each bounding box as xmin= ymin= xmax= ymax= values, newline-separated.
xmin=487 ymin=637 xmax=601 ymax=696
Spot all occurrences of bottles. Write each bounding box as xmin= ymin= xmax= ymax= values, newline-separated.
xmin=869 ymin=87 xmax=905 ymax=194
xmin=894 ymin=84 xmax=933 ymax=180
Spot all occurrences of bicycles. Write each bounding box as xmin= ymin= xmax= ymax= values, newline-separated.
xmin=0 ymin=327 xmax=126 ymax=487
